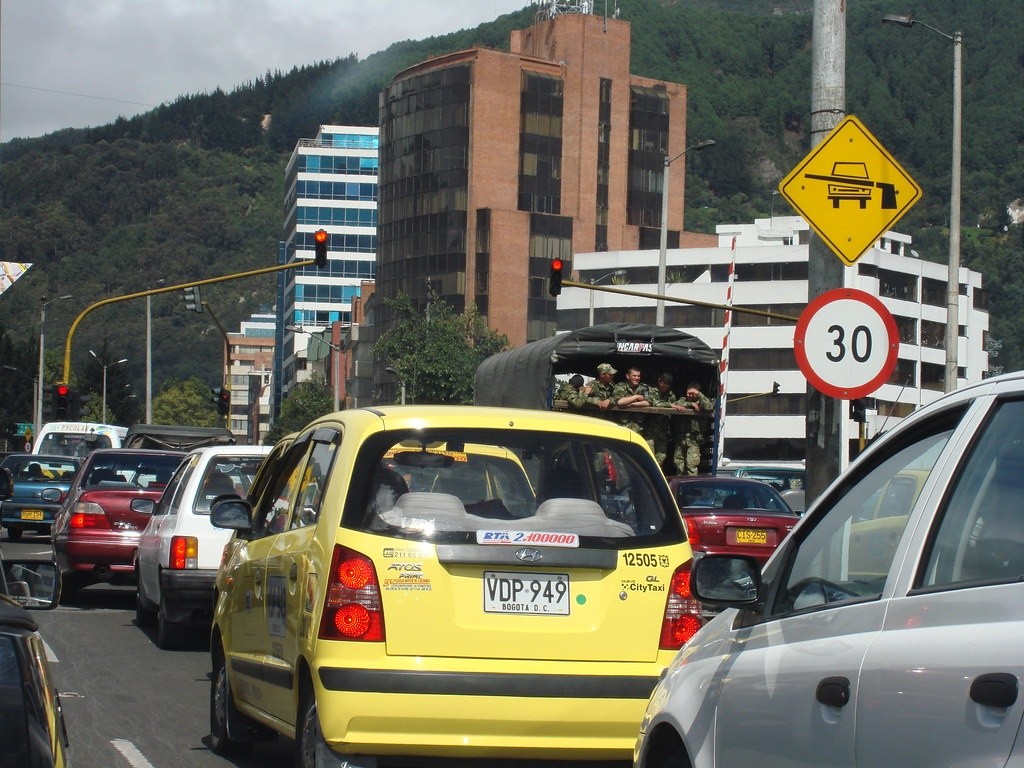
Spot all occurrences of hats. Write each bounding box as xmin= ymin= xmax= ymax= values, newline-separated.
xmin=597 ymin=363 xmax=618 ymax=375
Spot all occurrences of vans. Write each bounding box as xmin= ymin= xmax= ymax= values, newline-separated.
xmin=32 ymin=422 xmax=130 ymax=457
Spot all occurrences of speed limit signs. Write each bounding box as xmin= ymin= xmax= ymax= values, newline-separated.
xmin=794 ymin=289 xmax=900 ymax=401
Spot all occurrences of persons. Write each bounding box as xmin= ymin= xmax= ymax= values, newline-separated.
xmin=585 ymin=363 xmax=618 ymax=418
xmin=556 ymin=375 xmax=592 ymax=408
xmin=645 ymin=372 xmax=686 ymax=470
xmin=612 ymin=366 xmax=652 ymax=437
xmin=675 ymin=382 xmax=713 ymax=477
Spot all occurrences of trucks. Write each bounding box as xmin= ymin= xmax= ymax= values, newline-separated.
xmin=474 ymin=323 xmax=721 ymax=476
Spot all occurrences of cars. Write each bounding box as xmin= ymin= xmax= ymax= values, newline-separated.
xmin=208 ymin=401 xmax=695 ymax=768
xmin=0 ymin=445 xmax=276 ymax=768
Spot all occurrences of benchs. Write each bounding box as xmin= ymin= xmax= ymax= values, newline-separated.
xmin=383 ymin=492 xmax=635 ymax=538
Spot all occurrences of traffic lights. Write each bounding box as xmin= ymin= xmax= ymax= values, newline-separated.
xmin=56 ymin=385 xmax=67 ymax=419
xmin=315 ymin=230 xmax=327 ymax=269
xmin=184 ymin=287 xmax=196 ymax=310
xmin=853 ymin=396 xmax=866 ymax=422
xmin=550 ymin=260 xmax=562 ymax=295
xmin=211 ymin=388 xmax=230 ymax=415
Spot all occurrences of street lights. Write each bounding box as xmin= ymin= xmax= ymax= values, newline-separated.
xmin=385 ymin=367 xmax=406 ymax=405
xmin=87 ymin=349 xmax=127 ymax=424
xmin=656 ymin=138 xmax=716 ymax=327
xmin=145 ymin=277 xmax=165 ymax=425
xmin=589 ymin=270 xmax=627 ymax=326
xmin=285 ymin=325 xmax=343 ymax=412
xmin=884 ymin=11 xmax=964 ymax=392
xmin=3 ymin=365 xmax=37 ymax=446
xmin=38 ymin=293 xmax=74 ymax=432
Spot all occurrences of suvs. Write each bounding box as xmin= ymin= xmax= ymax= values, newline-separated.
xmin=122 ymin=425 xmax=237 ymax=450
xmin=632 ymin=372 xmax=1024 ymax=768
